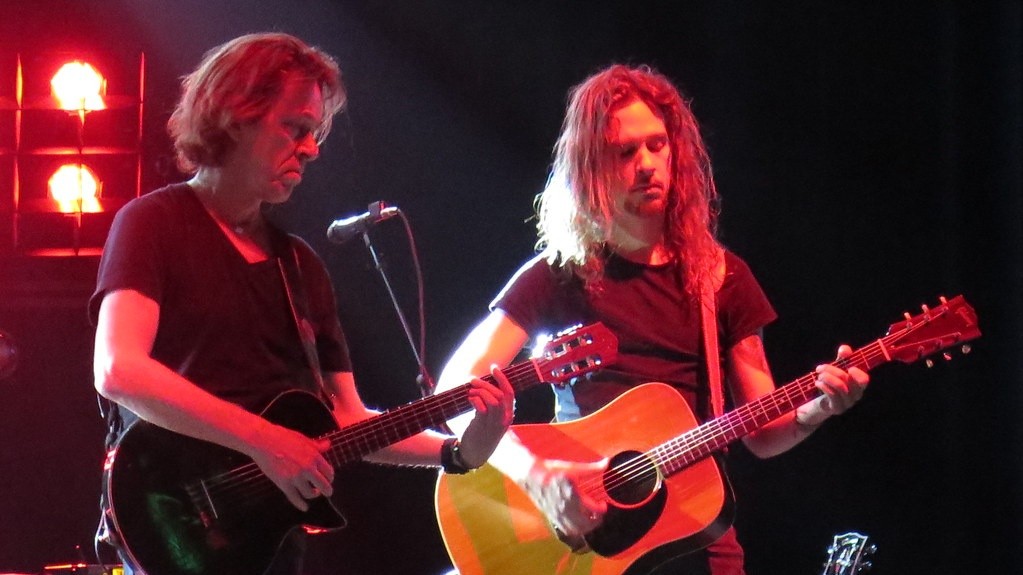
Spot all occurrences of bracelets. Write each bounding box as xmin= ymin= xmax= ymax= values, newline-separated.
xmin=441 ymin=437 xmax=472 ymax=474
xmin=793 ymin=410 xmax=820 ymax=432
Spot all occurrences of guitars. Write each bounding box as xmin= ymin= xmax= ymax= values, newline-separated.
xmin=101 ymin=320 xmax=620 ymax=575
xmin=433 ymin=294 xmax=982 ymax=575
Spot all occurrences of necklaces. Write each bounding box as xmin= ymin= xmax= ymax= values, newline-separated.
xmin=217 ymin=207 xmax=262 ymax=233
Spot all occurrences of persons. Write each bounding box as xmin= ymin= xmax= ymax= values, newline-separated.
xmin=433 ymin=64 xmax=870 ymax=575
xmin=89 ymin=34 xmax=515 ymax=575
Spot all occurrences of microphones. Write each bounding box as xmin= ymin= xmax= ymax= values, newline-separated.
xmin=327 ymin=206 xmax=398 ymax=245
